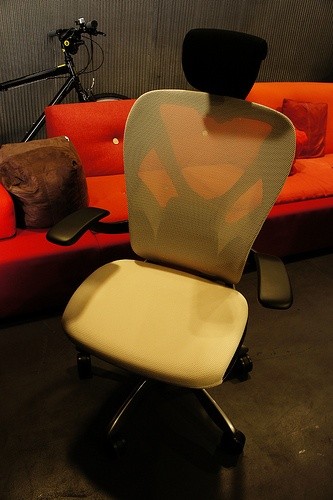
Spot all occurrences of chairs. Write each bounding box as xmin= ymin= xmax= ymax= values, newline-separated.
xmin=45 ymin=28 xmax=296 ymax=458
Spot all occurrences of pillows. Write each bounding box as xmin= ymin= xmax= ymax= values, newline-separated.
xmin=0 ymin=136 xmax=89 ymax=229
xmin=283 ymin=98 xmax=328 ymax=159
xmin=287 ymin=128 xmax=307 ymax=177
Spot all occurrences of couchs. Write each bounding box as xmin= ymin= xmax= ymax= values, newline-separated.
xmin=0 ymin=81 xmax=333 ymax=323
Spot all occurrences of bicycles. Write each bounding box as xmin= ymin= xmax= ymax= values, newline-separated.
xmin=0 ymin=17 xmax=131 ymax=145
